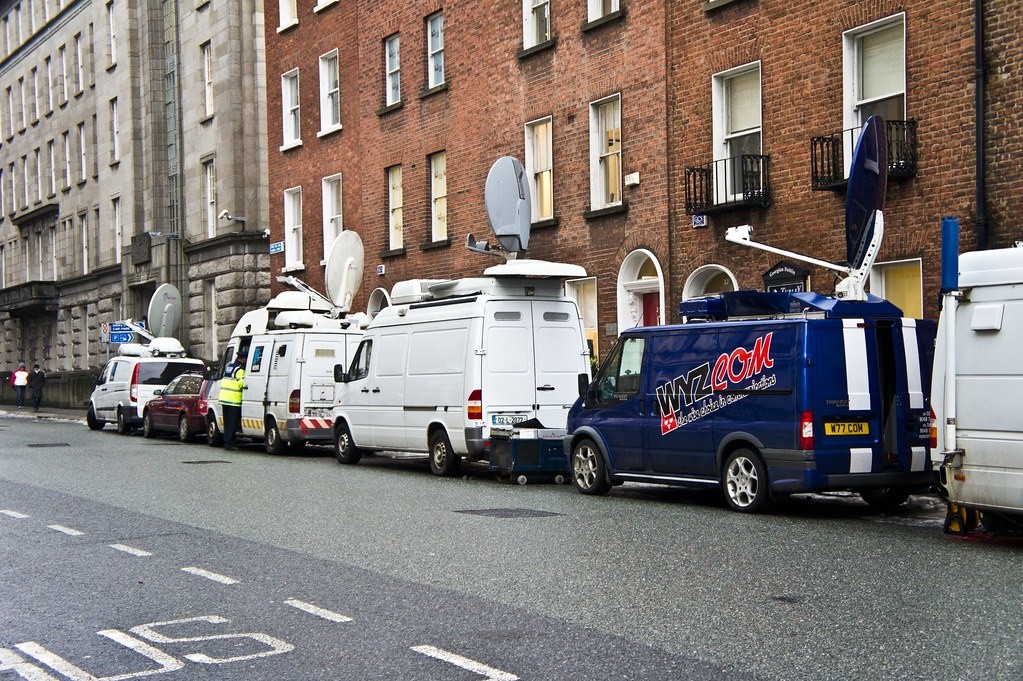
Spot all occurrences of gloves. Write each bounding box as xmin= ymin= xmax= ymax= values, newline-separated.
xmin=29 ymin=383 xmax=32 ymax=388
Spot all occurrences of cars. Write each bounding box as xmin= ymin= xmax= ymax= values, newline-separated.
xmin=142 ymin=370 xmax=218 ymax=443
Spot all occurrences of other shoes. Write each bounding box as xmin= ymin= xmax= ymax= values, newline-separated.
xmin=17 ymin=405 xmax=24 ymax=409
xmin=34 ymin=407 xmax=38 ymax=411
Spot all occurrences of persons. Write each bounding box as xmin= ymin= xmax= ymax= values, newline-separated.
xmin=11 ymin=363 xmax=45 ymax=413
xmin=217 ymin=352 xmax=249 ymax=450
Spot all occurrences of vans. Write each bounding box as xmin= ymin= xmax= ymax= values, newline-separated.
xmin=208 ymin=291 xmax=369 ymax=456
xmin=564 ymin=293 xmax=939 ymax=513
xmin=86 ymin=337 xmax=207 ymax=436
xmin=330 ymin=258 xmax=593 ymax=480
xmin=927 ymin=249 xmax=1023 ymax=533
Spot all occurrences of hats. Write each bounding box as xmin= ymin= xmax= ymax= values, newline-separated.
xmin=236 ymin=352 xmax=249 ymax=357
xmin=19 ymin=363 xmax=25 ymax=367
xmin=34 ymin=365 xmax=39 ymax=368
